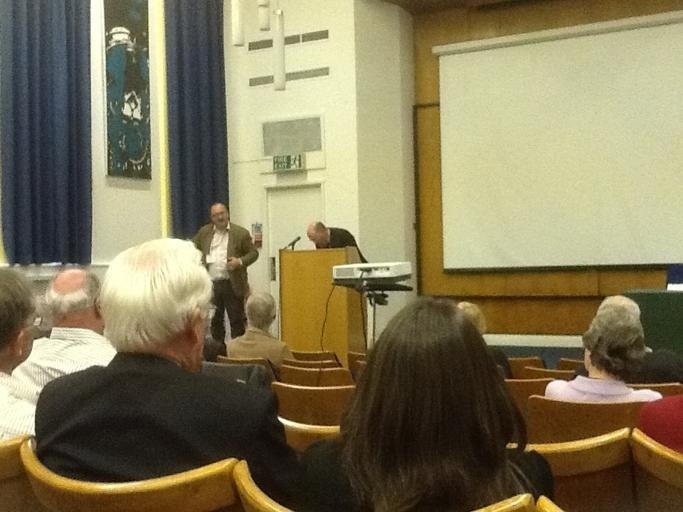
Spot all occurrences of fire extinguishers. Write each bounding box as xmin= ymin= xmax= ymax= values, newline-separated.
xmin=253 ymin=222 xmax=262 ymax=249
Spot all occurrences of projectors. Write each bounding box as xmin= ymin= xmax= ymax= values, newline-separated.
xmin=332 ymin=261 xmax=413 ymax=291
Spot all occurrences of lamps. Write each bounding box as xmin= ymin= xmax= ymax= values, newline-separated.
xmin=230 ymin=0 xmax=286 ymax=91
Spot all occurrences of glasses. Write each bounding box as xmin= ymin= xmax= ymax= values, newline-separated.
xmin=24 ymin=313 xmax=44 ymax=329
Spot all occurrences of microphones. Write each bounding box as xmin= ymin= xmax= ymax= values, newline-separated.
xmin=284 ymin=236 xmax=301 ymax=248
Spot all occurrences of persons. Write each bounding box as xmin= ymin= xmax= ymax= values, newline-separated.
xmin=456 ymin=302 xmax=512 ymax=379
xmin=225 ymin=288 xmax=298 ymax=361
xmin=8 ymin=265 xmax=117 ymax=402
xmin=290 ymin=294 xmax=555 ymax=511
xmin=1 ymin=266 xmax=44 ymax=437
xmin=32 ymin=238 xmax=298 ymax=510
xmin=198 ymin=336 xmax=227 ymax=363
xmin=545 ymin=308 xmax=661 ymax=401
xmin=306 ymin=221 xmax=367 ymax=264
xmin=190 ymin=201 xmax=261 ymax=344
xmin=570 ymin=295 xmax=680 ymax=381
